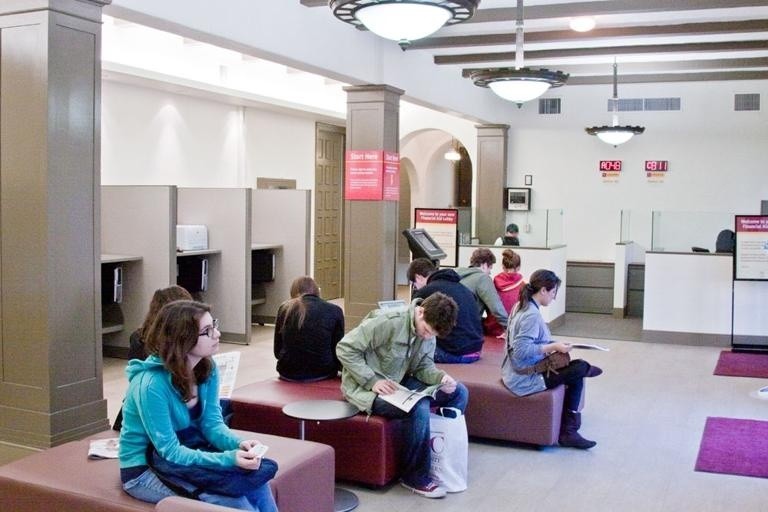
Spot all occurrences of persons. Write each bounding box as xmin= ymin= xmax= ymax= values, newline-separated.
xmin=485 ymin=249 xmax=526 ymax=337
xmin=406 ymin=258 xmax=484 ymax=364
xmin=118 ymin=299 xmax=279 ymax=512
xmin=494 ymin=223 xmax=520 ymax=246
xmin=454 ymin=248 xmax=509 ymax=339
xmin=273 ymin=276 xmax=345 ymax=385
xmin=500 ymin=268 xmax=603 ymax=449
xmin=112 ymin=286 xmax=232 ymax=432
xmin=334 ymin=292 xmax=468 ymax=498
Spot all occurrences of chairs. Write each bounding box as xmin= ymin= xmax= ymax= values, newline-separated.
xmin=716 ymin=229 xmax=735 ymax=253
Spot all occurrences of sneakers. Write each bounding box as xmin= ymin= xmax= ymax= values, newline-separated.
xmin=400 ymin=481 xmax=447 ymax=499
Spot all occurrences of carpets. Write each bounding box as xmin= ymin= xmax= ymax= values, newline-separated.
xmin=713 ymin=351 xmax=768 ymax=381
xmin=694 ymin=416 xmax=768 ymax=479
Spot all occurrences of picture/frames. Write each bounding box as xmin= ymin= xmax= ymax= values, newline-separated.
xmin=507 ymin=187 xmax=531 ymax=212
xmin=525 ymin=175 xmax=533 ymax=186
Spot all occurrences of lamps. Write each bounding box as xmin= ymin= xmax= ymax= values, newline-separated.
xmin=469 ymin=0 xmax=570 ymax=110
xmin=327 ymin=0 xmax=480 ymax=52
xmin=444 ymin=136 xmax=461 ymax=161
xmin=584 ymin=63 xmax=646 ymax=148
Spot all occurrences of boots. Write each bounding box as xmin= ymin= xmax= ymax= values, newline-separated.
xmin=585 ymin=365 xmax=603 ymax=379
xmin=558 ymin=408 xmax=597 ymax=450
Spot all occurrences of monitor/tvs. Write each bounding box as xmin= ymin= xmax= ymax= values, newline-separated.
xmin=403 ymin=228 xmax=447 ymax=260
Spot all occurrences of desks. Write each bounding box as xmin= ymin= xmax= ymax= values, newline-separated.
xmin=101 ymin=254 xmax=142 ymax=334
xmin=176 ymin=249 xmax=221 ymax=256
xmin=252 ymin=243 xmax=283 ymax=306
xmin=282 ymin=399 xmax=360 ymax=512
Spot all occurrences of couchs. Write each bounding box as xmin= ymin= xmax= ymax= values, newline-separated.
xmin=432 ymin=336 xmax=585 ymax=448
xmin=221 ymin=375 xmax=437 ymax=489
xmin=0 ymin=429 xmax=335 ymax=511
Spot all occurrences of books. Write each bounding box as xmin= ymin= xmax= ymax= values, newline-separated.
xmin=571 ymin=340 xmax=611 ymax=354
xmin=376 ymin=374 xmax=452 ymax=415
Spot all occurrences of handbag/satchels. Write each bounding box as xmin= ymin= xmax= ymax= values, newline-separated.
xmin=505 ymin=304 xmax=570 ymax=375
xmin=427 ymin=406 xmax=469 ymax=494
xmin=145 ymin=427 xmax=279 ymax=501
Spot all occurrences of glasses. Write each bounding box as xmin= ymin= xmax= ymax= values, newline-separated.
xmin=410 ymin=281 xmax=416 ymax=292
xmin=197 ymin=319 xmax=220 ymax=338
